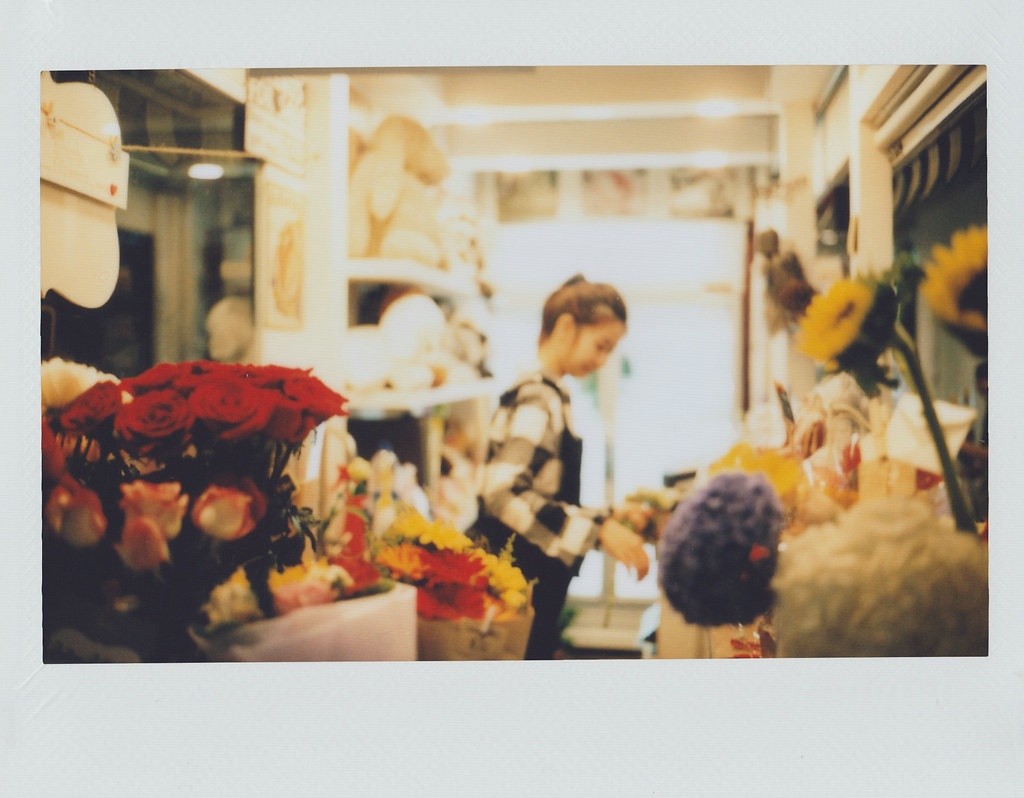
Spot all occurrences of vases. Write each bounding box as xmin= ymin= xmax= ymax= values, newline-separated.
xmin=418 ymin=603 xmax=535 ymax=661
xmin=225 ymin=580 xmax=419 ymax=662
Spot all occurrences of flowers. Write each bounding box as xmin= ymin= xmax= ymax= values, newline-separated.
xmin=653 ymin=226 xmax=990 ymax=656
xmin=43 ymin=224 xmax=537 ymax=662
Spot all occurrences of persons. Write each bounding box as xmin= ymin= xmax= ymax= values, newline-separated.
xmin=466 ymin=275 xmax=653 ymax=661
